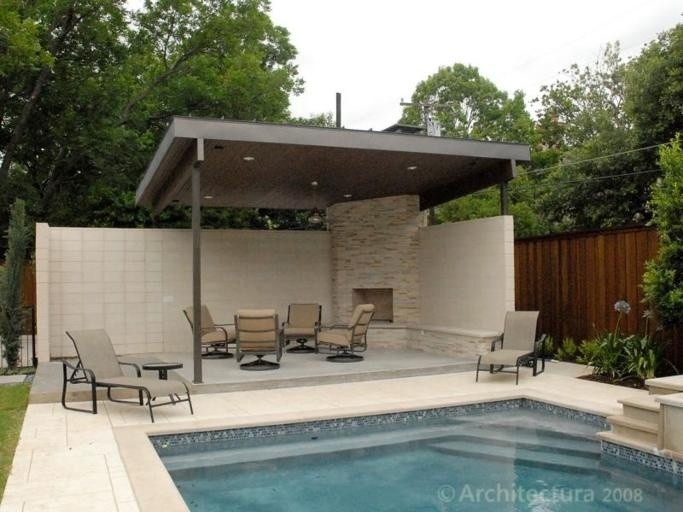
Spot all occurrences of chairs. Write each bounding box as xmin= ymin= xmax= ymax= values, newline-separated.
xmin=62 ymin=329 xmax=194 ymax=423
xmin=315 ymin=304 xmax=376 ymax=362
xmin=184 ymin=304 xmax=236 ymax=360
xmin=234 ymin=309 xmax=282 ymax=370
xmin=282 ymin=302 xmax=322 ymax=353
xmin=475 ymin=312 xmax=547 ymax=385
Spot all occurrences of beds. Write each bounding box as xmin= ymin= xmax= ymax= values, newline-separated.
xmin=142 ymin=362 xmax=183 ymax=404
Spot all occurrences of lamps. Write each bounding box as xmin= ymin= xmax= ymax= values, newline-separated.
xmin=307 ymin=182 xmax=323 ymax=223
